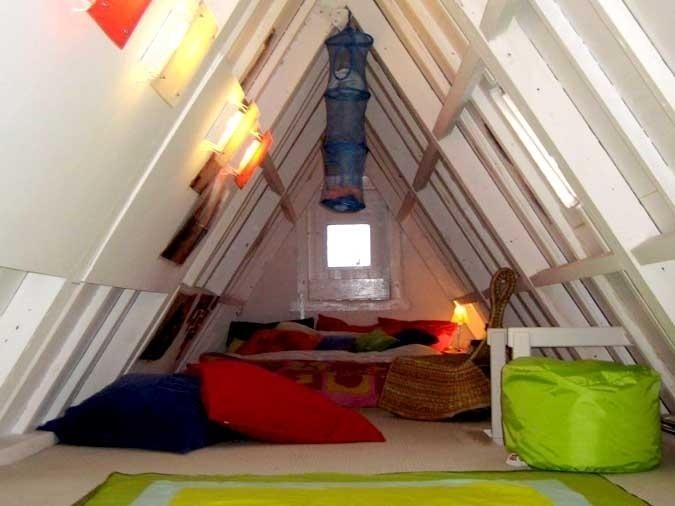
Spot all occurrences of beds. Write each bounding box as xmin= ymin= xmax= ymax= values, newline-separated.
xmin=208 ymin=352 xmax=444 ymax=408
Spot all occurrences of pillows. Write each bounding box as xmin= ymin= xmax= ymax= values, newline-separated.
xmin=234 ymin=329 xmax=325 ymax=356
xmin=200 ymin=353 xmax=387 ymax=443
xmin=316 ymin=313 xmax=349 ymax=331
xmin=353 ymin=328 xmax=397 ymax=352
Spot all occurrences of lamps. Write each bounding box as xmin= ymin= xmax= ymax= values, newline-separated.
xmin=450 ymin=305 xmax=469 ymax=353
xmin=200 ymin=99 xmax=262 ymax=159
xmin=224 ymin=129 xmax=273 ymax=190
xmin=139 ymin=0 xmax=219 ymax=107
xmin=85 ymin=0 xmax=155 ymax=49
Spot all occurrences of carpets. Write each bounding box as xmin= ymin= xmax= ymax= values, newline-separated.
xmin=71 ymin=468 xmax=650 ymax=506
